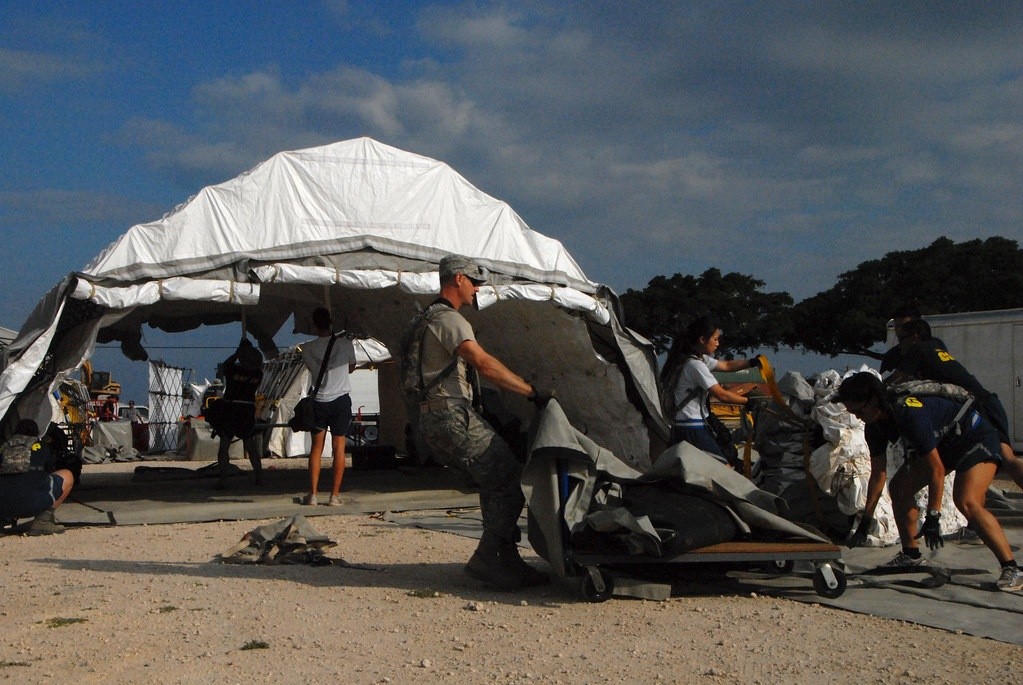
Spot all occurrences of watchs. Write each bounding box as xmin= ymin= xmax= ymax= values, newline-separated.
xmin=927 ymin=509 xmax=942 ymax=517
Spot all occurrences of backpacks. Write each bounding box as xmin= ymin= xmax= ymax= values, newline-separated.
xmin=656 ymin=356 xmax=703 ymax=416
xmin=0 ymin=434 xmax=38 ymax=475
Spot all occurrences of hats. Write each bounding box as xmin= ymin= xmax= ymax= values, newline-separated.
xmin=239 ymin=347 xmax=265 ymax=371
xmin=439 ymin=254 xmax=487 ymax=283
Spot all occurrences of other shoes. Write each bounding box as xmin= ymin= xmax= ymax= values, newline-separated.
xmin=252 ymin=468 xmax=265 ymax=485
xmin=29 ymin=512 xmax=65 ymax=536
xmin=300 ymin=493 xmax=317 ymax=506
xmin=463 ymin=550 xmax=523 ymax=591
xmin=328 ymin=495 xmax=344 ymax=507
xmin=499 ymin=549 xmax=537 ymax=575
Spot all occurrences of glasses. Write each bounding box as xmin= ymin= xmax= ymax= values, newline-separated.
xmin=897 ymin=333 xmax=921 ymax=343
xmin=845 ymin=399 xmax=870 ymax=418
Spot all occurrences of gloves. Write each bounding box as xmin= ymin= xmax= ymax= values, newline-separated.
xmin=846 ymin=516 xmax=873 ymax=550
xmin=745 ymin=398 xmax=759 ymax=412
xmin=528 ymin=385 xmax=558 ymax=410
xmin=914 ymin=516 xmax=943 ymax=551
xmin=749 ymin=354 xmax=762 ymax=368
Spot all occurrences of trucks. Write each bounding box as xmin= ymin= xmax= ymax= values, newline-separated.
xmin=90 ymin=402 xmax=148 ymax=425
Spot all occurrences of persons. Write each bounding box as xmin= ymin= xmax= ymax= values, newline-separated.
xmin=399 ymin=255 xmax=557 ymax=590
xmin=299 ymin=306 xmax=356 ymax=506
xmin=840 ymin=372 xmax=1023 ymax=592
xmin=1 ymin=419 xmax=73 ymax=535
xmin=659 ymin=314 xmax=761 ymax=474
xmin=202 ymin=379 xmax=224 ymax=413
xmin=101 ymin=395 xmax=119 ymax=422
xmin=887 ymin=318 xmax=1023 ymax=544
xmin=124 ymin=400 xmax=143 ymax=424
xmin=215 ymin=339 xmax=266 ymax=488
xmin=879 ymin=304 xmax=947 ymax=370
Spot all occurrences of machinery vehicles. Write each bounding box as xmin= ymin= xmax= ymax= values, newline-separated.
xmin=82 ymin=360 xmax=122 ymax=403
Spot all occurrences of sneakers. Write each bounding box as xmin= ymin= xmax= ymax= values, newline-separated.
xmin=942 ymin=526 xmax=984 ymax=545
xmin=992 ymin=566 xmax=1023 ymax=591
xmin=882 ymin=551 xmax=927 ymax=568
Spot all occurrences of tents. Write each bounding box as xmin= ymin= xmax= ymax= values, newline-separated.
xmin=1 ymin=136 xmax=666 ymax=449
xmin=267 ymin=336 xmax=389 ymax=458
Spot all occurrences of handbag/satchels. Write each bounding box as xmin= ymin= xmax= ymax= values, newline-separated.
xmin=289 ymin=396 xmax=330 ymax=432
xmin=204 ymin=398 xmax=233 ymax=430
xmin=704 ymin=413 xmax=733 ymax=446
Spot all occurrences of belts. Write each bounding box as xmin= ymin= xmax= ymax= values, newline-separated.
xmin=419 ymin=397 xmax=468 ymax=415
